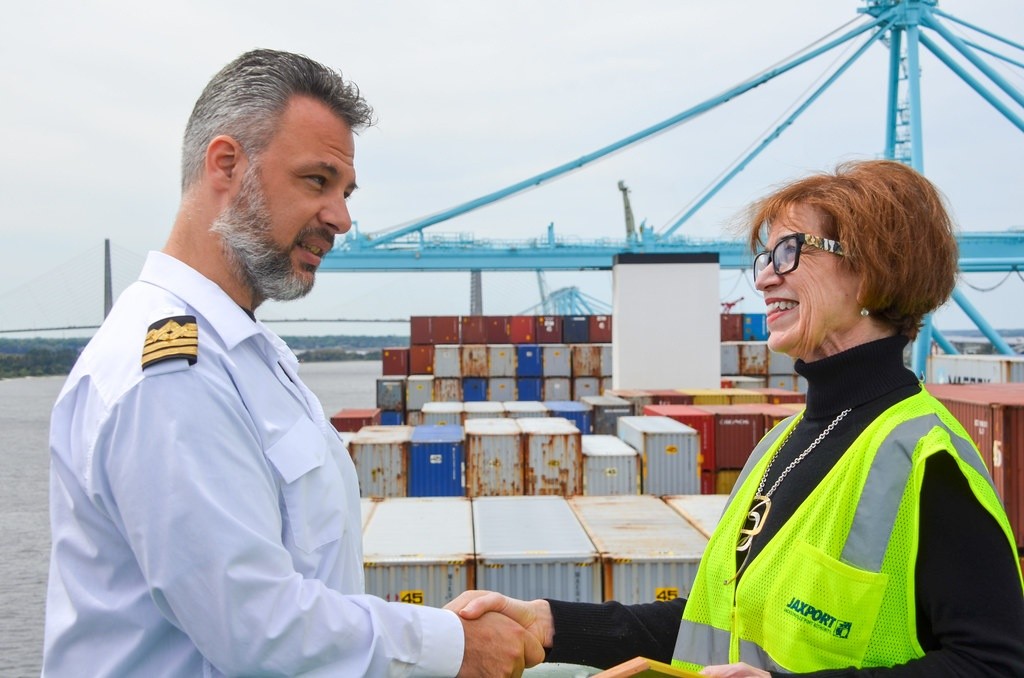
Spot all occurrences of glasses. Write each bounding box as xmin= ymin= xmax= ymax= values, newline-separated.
xmin=754 ymin=232 xmax=861 ymax=283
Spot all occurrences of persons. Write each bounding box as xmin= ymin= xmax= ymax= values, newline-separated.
xmin=41 ymin=47 xmax=547 ymax=677
xmin=442 ymin=157 xmax=1024 ymax=677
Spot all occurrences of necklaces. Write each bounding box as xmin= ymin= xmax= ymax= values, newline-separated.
xmin=736 ymin=405 xmax=852 ymax=554
